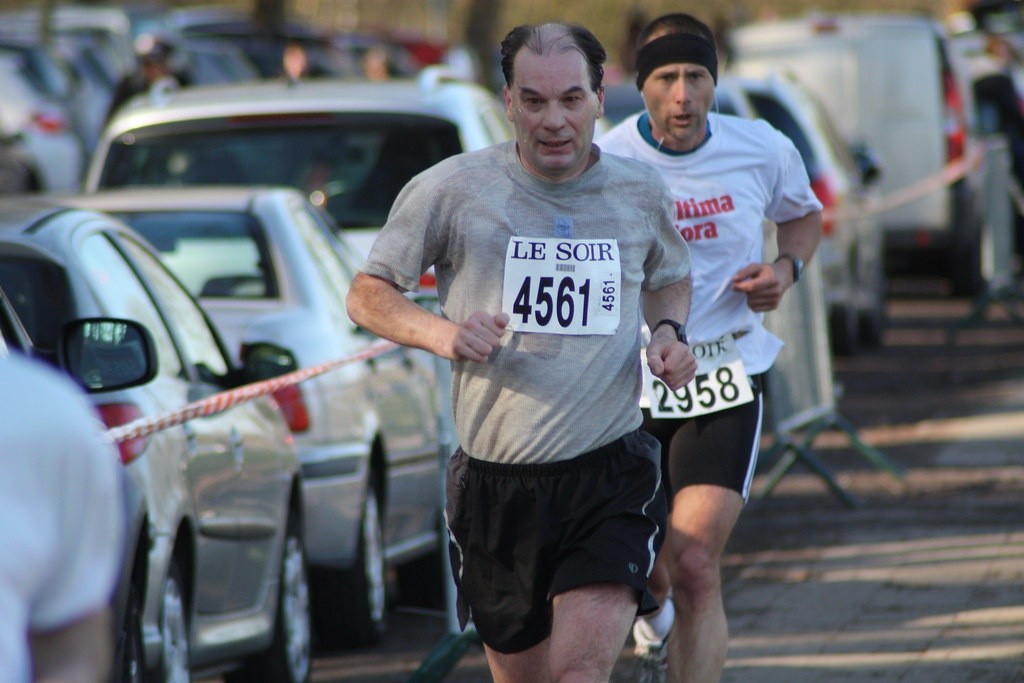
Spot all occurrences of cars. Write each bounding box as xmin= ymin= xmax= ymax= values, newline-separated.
xmin=0 ymin=9 xmax=1023 ymax=359
xmin=38 ymin=193 xmax=455 ymax=644
xmin=0 ymin=190 xmax=322 ymax=683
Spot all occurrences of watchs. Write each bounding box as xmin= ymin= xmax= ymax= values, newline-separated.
xmin=653 ymin=319 xmax=689 ymax=345
xmin=775 ymin=253 xmax=803 ymax=282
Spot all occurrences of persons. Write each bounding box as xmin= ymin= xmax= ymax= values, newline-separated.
xmin=106 ymin=34 xmax=191 ymax=185
xmin=271 ymin=40 xmax=328 ymax=79
xmin=0 ymin=351 xmax=126 ymax=683
xmin=594 ymin=12 xmax=824 ymax=683
xmin=361 ymin=44 xmax=391 ymax=82
xmin=346 ymin=25 xmax=698 ymax=683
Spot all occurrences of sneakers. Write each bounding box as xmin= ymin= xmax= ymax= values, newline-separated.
xmin=626 ymin=596 xmax=678 ymax=683
